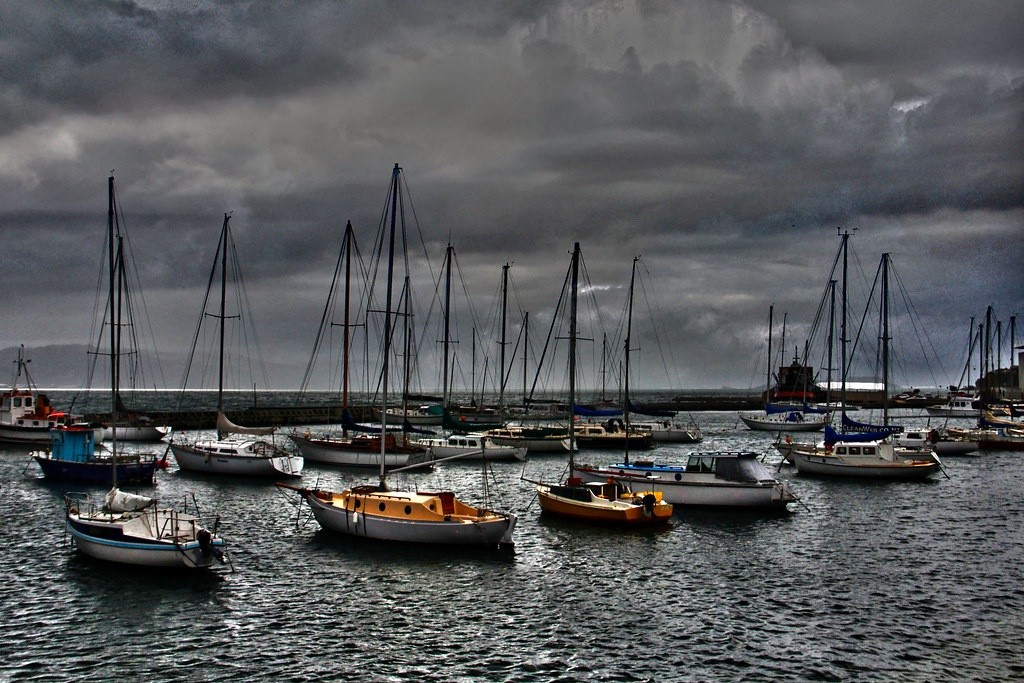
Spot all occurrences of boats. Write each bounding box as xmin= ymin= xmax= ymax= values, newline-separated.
xmin=763 ymin=338 xmax=830 ymax=405
xmin=816 ymin=402 xmax=859 ymax=412
xmin=776 ymin=225 xmax=1024 ymax=481
xmin=927 ymin=390 xmax=982 ymax=417
xmin=0 ymin=345 xmax=106 ymax=444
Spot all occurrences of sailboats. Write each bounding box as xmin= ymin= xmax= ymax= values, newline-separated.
xmin=520 ymin=240 xmax=673 ymax=526
xmin=574 ymin=256 xmax=702 ymax=447
xmin=26 ymin=232 xmax=161 ymax=488
xmin=736 ymin=304 xmax=830 ymax=432
xmin=280 ymin=159 xmax=517 ymax=550
xmin=287 ymin=220 xmax=580 ymax=469
xmin=584 ymin=255 xmax=800 ymax=514
xmin=168 ymin=211 xmax=306 ymax=481
xmin=65 ymin=167 xmax=227 ymax=569
